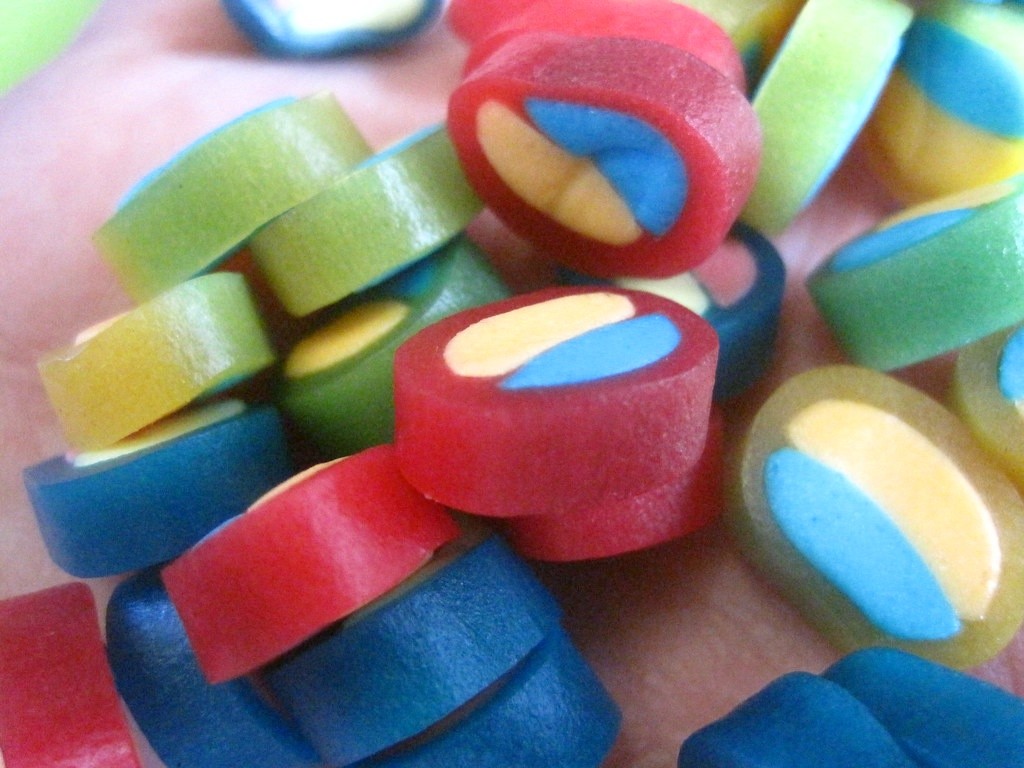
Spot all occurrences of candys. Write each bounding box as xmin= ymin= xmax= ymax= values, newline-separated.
xmin=0 ymin=0 xmax=1024 ymax=768
